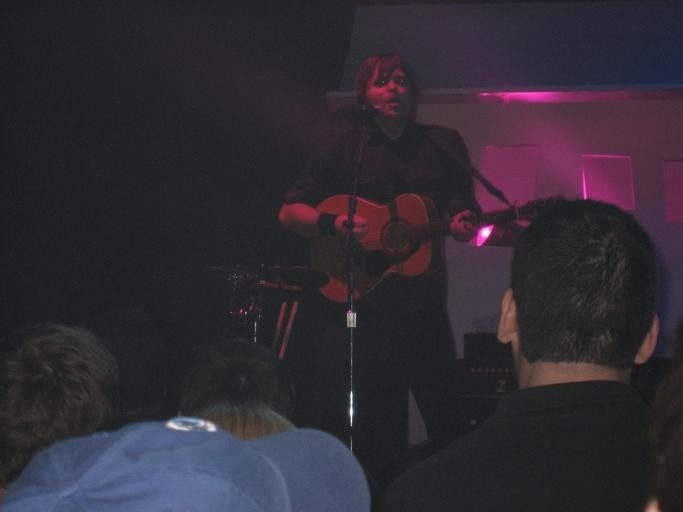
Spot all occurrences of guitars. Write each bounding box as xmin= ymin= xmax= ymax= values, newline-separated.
xmin=310 ymin=192 xmax=565 ymax=304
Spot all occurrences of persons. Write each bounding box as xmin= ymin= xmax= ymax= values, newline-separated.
xmin=1 ymin=323 xmax=370 ymax=511
xmin=278 ymin=54 xmax=482 ymax=485
xmin=627 ymin=367 xmax=682 ymax=512
xmin=374 ymin=195 xmax=659 ymax=512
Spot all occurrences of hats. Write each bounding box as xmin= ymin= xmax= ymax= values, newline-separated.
xmin=1 ymin=415 xmax=372 ymax=512
